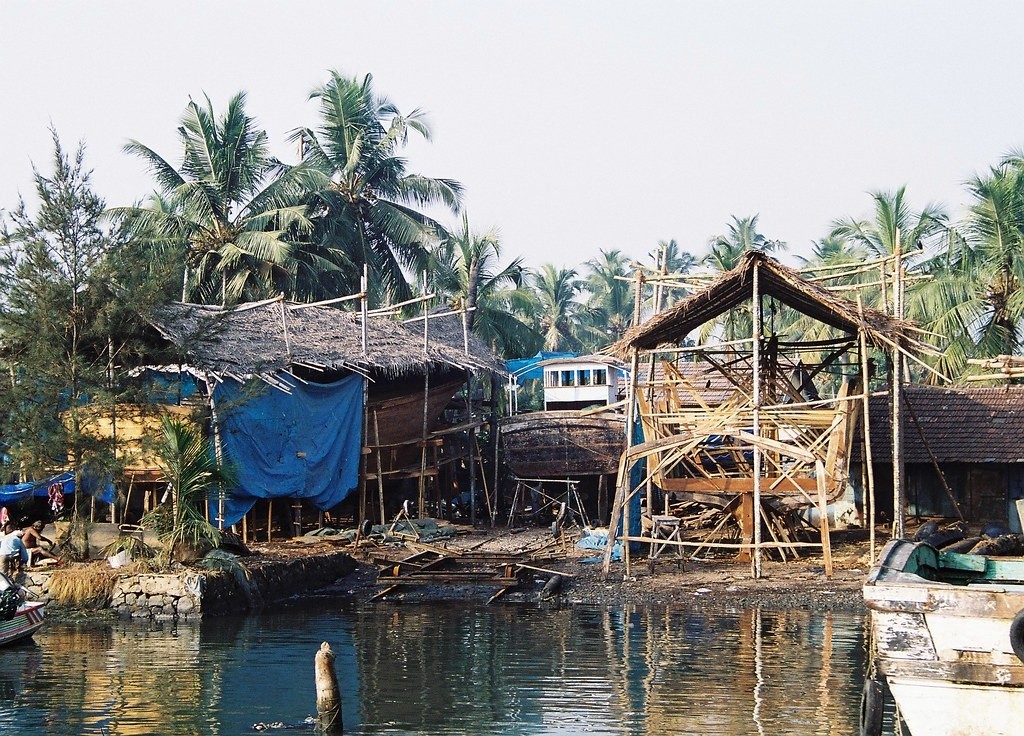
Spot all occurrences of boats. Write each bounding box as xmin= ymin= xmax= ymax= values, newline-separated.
xmin=1 ymin=572 xmax=45 ymax=647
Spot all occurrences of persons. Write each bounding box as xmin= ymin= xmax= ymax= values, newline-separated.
xmin=22 ymin=520 xmax=60 ymax=560
xmin=0 ymin=502 xmax=28 ymax=575
xmin=47 ymin=482 xmax=65 ymax=520
